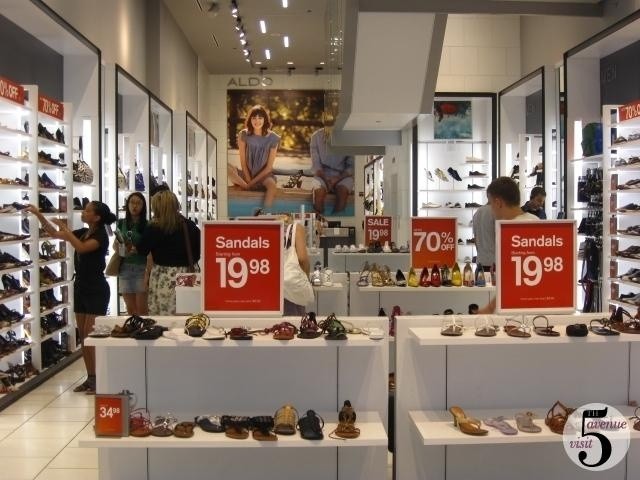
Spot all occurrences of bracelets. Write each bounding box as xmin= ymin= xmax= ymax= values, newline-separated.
xmin=40 ymin=222 xmax=47 ymax=229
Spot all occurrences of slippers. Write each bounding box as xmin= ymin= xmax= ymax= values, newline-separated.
xmin=515 ymin=411 xmax=543 ymax=434
xmin=174 ymin=420 xmax=196 ymax=437
xmin=483 ymin=416 xmax=517 ymax=435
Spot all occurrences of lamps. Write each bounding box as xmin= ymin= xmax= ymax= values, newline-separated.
xmin=230 ymin=0 xmax=252 ymax=63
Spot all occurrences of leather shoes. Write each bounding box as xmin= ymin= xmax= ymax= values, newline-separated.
xmin=73 ymin=197 xmax=89 ymax=210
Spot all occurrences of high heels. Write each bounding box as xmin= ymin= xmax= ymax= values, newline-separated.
xmin=282 ymin=169 xmax=303 ymax=189
xmin=310 ymin=260 xmax=496 ymax=288
xmin=448 ymin=405 xmax=489 ymax=436
xmin=0 ymin=119 xmax=71 ymax=393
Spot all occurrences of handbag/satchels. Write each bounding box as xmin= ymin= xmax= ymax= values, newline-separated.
xmin=73 ymin=136 xmax=93 ymax=184
xmin=106 ymin=220 xmax=124 ymax=276
xmin=577 ymin=123 xmax=602 ymax=258
xmin=282 ymin=221 xmax=316 ymax=309
xmin=118 ymin=156 xmax=169 ymax=192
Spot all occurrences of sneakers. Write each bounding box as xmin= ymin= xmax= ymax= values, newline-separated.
xmin=614 ymin=133 xmax=640 ymax=307
xmin=424 ymin=152 xmax=498 ymax=243
xmin=333 ymin=241 xmax=409 ymax=254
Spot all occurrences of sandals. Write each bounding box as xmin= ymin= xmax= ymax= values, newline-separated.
xmin=545 ymin=401 xmax=576 ymax=436
xmin=129 ymin=407 xmax=152 ymax=436
xmin=440 ymin=307 xmax=639 ymax=338
xmin=194 ymin=413 xmax=224 ymax=433
xmin=74 ymin=381 xmax=95 ymax=393
xmin=295 ymin=409 xmax=325 ymax=440
xmin=222 ymin=412 xmax=278 ymax=441
xmin=89 ymin=311 xmax=388 ymax=342
xmin=274 ymin=403 xmax=298 ymax=437
xmin=335 ymin=400 xmax=361 ymax=440
xmin=152 ymin=413 xmax=176 ymax=437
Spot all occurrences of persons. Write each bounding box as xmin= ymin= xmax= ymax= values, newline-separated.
xmin=278 ymin=215 xmax=315 ymax=323
xmin=473 ymin=175 xmax=542 ymax=315
xmin=311 ymin=117 xmax=354 ymax=213
xmin=228 ymin=105 xmax=281 ymax=207
xmin=23 ymin=200 xmax=111 ymax=394
xmin=521 ymin=186 xmax=548 ymax=219
xmin=134 ymin=191 xmax=202 ymax=315
xmin=474 ymin=195 xmax=493 ymax=280
xmin=105 ymin=192 xmax=150 ymax=316
